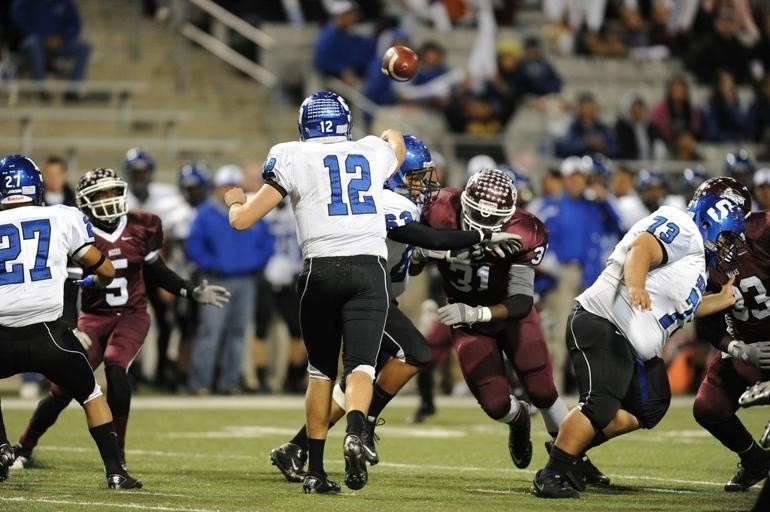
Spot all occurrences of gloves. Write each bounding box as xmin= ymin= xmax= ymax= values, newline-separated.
xmin=481 ymin=228 xmax=524 ymax=258
xmin=439 ymin=303 xmax=483 ymax=325
xmin=193 ymin=279 xmax=232 ymax=307
xmin=71 ymin=274 xmax=97 ymax=291
xmin=732 ymin=339 xmax=770 ymax=368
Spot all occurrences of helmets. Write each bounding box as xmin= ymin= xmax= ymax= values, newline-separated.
xmin=125 ymin=148 xmax=154 ymax=184
xmin=460 ymin=167 xmax=517 ymax=232
xmin=179 ymin=161 xmax=213 ymax=205
xmin=384 ymin=135 xmax=441 ymax=207
xmin=691 ymin=196 xmax=749 ymax=271
xmin=690 ymin=176 xmax=752 ymax=216
xmin=297 ymin=90 xmax=353 ymax=143
xmin=75 ymin=168 xmax=128 ymax=220
xmin=0 ymin=155 xmax=47 ymax=212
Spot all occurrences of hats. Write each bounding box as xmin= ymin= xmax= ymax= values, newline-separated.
xmin=561 ymin=156 xmax=592 ymax=178
xmin=213 ymin=166 xmax=243 ymax=188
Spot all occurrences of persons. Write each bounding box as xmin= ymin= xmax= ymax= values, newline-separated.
xmin=247 ymin=191 xmax=303 ymax=392
xmin=417 ymin=35 xmax=468 ymax=104
xmin=224 ymin=90 xmax=406 ymax=496
xmin=13 ymin=166 xmax=232 ymax=474
xmin=599 ymin=164 xmax=650 ymax=231
xmin=393 ymin=169 xmax=570 ymax=469
xmin=514 ymin=31 xmax=562 ymax=93
xmin=547 ymin=0 xmax=770 ymax=79
xmin=452 ymin=38 xmax=528 ymax=132
xmin=543 ymin=155 xmax=603 ymax=388
xmin=0 ymin=153 xmax=144 ymax=490
xmin=552 ymin=93 xmax=618 ymax=157
xmin=719 ymin=147 xmax=757 ymax=180
xmin=753 ymin=71 xmax=770 ymax=143
xmin=650 ymin=73 xmax=708 ymax=161
xmin=312 ymin=0 xmax=372 ymax=82
xmin=704 ymin=70 xmax=750 ymax=144
xmin=611 ymin=91 xmax=663 ymax=159
xmin=37 ymin=158 xmax=80 ymax=331
xmin=694 ymin=175 xmax=770 ymax=492
xmin=162 ymin=163 xmax=213 ymax=368
xmin=189 ymin=165 xmax=276 ymax=395
xmin=0 ymin=0 xmax=92 ymax=102
xmin=271 ymin=135 xmax=523 ymax=481
xmin=123 ymin=147 xmax=199 ymax=381
xmin=532 ymin=195 xmax=747 ymax=499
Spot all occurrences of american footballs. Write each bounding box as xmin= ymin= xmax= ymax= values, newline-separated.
xmin=381 ymin=45 xmax=420 ymax=83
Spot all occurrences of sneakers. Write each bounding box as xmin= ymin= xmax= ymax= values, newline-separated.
xmin=534 ymin=470 xmax=581 ymax=499
xmin=509 ymin=401 xmax=533 ymax=468
xmin=738 ymin=380 xmax=770 ymax=407
xmin=271 ymin=442 xmax=307 ymax=481
xmin=343 ymin=430 xmax=368 ymax=491
xmin=360 ymin=417 xmax=386 ymax=464
xmin=10 ymin=443 xmax=33 ymax=462
xmin=408 ymin=402 xmax=435 ymax=424
xmin=581 ymin=455 xmax=611 ymax=485
xmin=546 ymin=438 xmax=587 ymax=490
xmin=723 ymin=450 xmax=770 ymax=492
xmin=303 ymin=472 xmax=341 ymax=493
xmin=108 ymin=471 xmax=143 ymax=491
xmin=758 ymin=421 xmax=770 ymax=447
xmin=0 ymin=443 xmax=14 ymax=479
